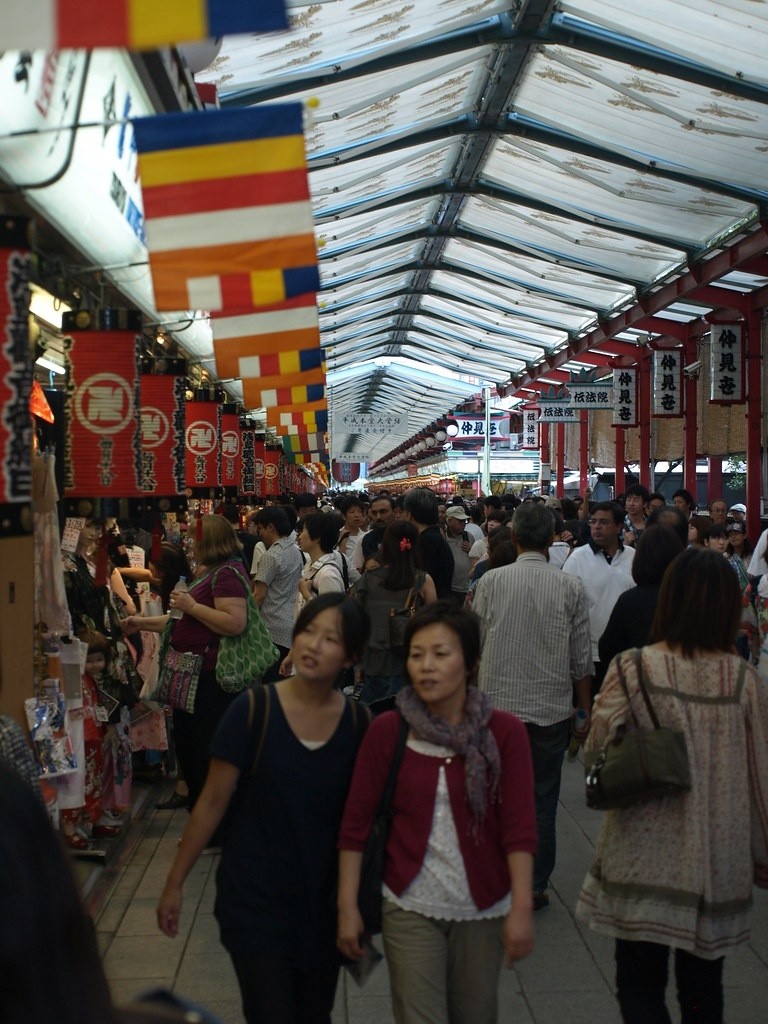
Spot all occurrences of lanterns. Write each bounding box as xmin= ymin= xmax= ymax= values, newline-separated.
xmin=332 ymin=457 xmax=360 ymax=486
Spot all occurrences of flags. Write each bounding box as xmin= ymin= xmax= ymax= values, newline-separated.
xmin=132 ymin=100 xmax=319 ymax=278
xmin=150 ymin=265 xmax=330 ymax=475
xmin=54 ymin=0 xmax=291 ymax=52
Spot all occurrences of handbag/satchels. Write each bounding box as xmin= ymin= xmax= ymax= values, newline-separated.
xmin=211 ymin=565 xmax=281 ymax=694
xmin=583 ymin=648 xmax=692 ymax=811
xmin=386 ymin=574 xmax=422 ymax=654
xmin=350 ymin=716 xmax=410 ymax=933
xmin=149 ymin=648 xmax=202 ymax=714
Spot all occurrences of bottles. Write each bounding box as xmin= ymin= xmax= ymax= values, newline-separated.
xmin=170 ymin=576 xmax=188 ymax=620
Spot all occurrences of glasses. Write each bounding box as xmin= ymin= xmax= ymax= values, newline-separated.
xmin=588 ymin=519 xmax=617 ymax=527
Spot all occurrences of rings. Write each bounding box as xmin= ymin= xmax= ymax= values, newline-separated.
xmin=170 ymin=599 xmax=174 ymax=604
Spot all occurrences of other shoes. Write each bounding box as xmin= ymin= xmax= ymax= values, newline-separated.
xmin=533 ymin=892 xmax=548 ymax=910
xmin=155 ymin=792 xmax=189 ymax=809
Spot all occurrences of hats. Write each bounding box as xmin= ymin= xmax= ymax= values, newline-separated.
xmin=730 ymin=504 xmax=747 ymax=514
xmin=727 ymin=522 xmax=745 ymax=533
xmin=545 ymin=498 xmax=562 ymax=509
xmin=447 ymin=506 xmax=471 ymax=520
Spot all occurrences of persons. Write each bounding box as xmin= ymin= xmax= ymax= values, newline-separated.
xmin=120 ymin=514 xmax=249 ymax=855
xmin=471 ymin=502 xmax=596 ymax=909
xmin=573 ymin=546 xmax=768 ymax=1024
xmin=65 ymin=503 xmax=108 ymax=635
xmin=0 ymin=758 xmax=117 ymax=1024
xmin=214 ymin=487 xmax=768 ymax=716
xmin=336 ymin=599 xmax=538 ymax=1023
xmin=115 ymin=540 xmax=196 ymax=611
xmin=156 ymin=592 xmax=392 ymax=1024
xmin=59 ymin=629 xmax=134 ymax=849
